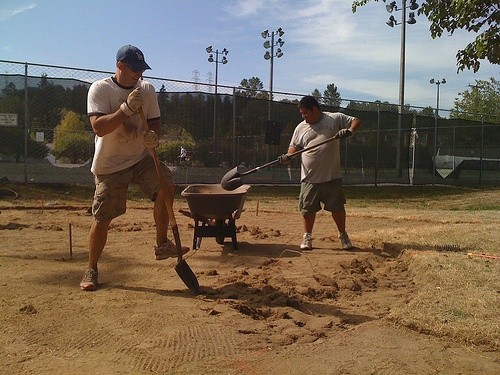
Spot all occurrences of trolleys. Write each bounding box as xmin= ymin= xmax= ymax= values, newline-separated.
xmin=178 ymin=183 xmax=250 ymax=250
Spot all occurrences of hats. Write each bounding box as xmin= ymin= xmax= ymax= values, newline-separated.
xmin=116 ymin=44 xmax=152 ymax=71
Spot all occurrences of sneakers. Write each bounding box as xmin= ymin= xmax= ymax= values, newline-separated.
xmin=154 ymin=239 xmax=190 ymax=260
xmin=80 ymin=267 xmax=99 ymax=290
xmin=300 ymin=233 xmax=312 ymax=249
xmin=338 ymin=233 xmax=352 ymax=248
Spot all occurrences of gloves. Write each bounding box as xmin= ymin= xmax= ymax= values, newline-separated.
xmin=143 ymin=129 xmax=159 ymax=149
xmin=277 ymin=153 xmax=293 ymax=164
xmin=120 ymin=86 xmax=145 ymax=116
xmin=335 ymin=128 xmax=353 ymax=140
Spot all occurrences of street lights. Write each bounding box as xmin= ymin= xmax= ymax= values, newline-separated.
xmin=385 ymin=0 xmax=419 ymax=178
xmin=430 ymin=78 xmax=447 ymax=189
xmin=205 ymin=45 xmax=229 ymax=138
xmin=261 ymin=26 xmax=285 ymax=163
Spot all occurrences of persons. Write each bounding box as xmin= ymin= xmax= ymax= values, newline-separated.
xmin=180 ymin=144 xmax=190 ymax=168
xmin=79 ymin=45 xmax=190 ymax=292
xmin=277 ymin=96 xmax=362 ymax=250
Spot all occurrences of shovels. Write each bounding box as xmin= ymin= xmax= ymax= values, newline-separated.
xmin=139 ymin=106 xmax=201 ymax=295
xmin=220 ymin=137 xmax=335 ymax=191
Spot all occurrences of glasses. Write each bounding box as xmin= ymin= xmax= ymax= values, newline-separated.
xmin=126 ymin=65 xmax=145 ymax=72
